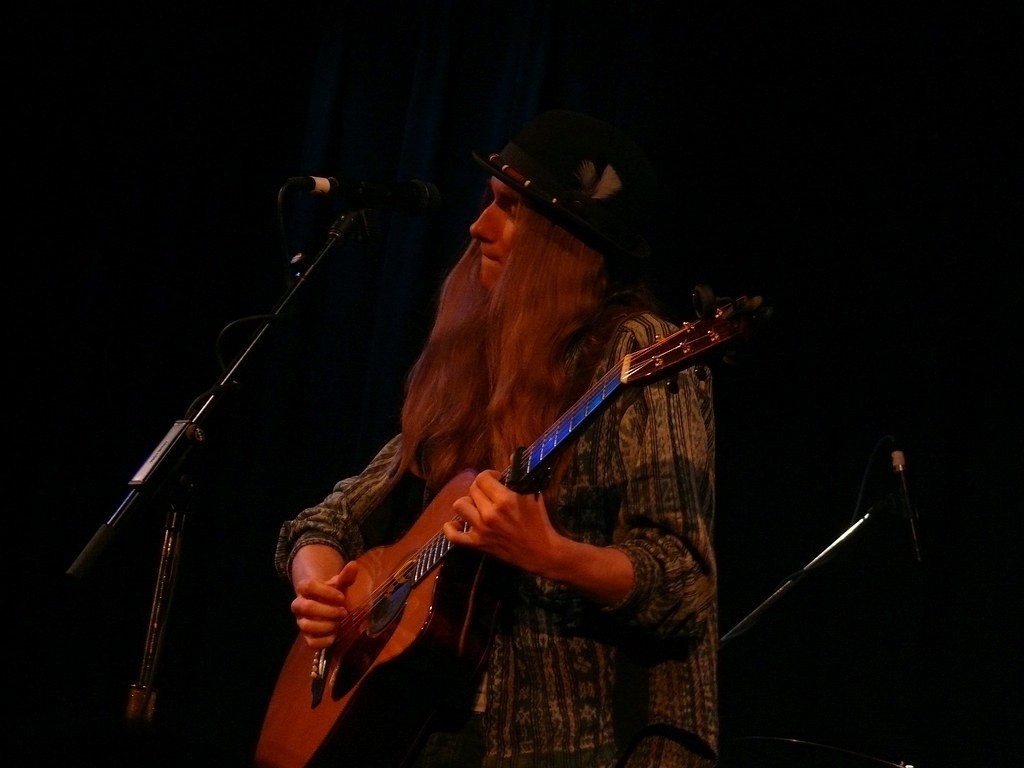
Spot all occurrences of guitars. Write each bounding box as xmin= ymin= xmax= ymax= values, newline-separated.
xmin=253 ymin=291 xmax=751 ymax=768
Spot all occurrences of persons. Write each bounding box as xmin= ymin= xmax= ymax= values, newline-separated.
xmin=274 ymin=115 xmax=721 ymax=768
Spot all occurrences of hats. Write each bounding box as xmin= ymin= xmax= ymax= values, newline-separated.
xmin=469 ymin=125 xmax=658 ymax=270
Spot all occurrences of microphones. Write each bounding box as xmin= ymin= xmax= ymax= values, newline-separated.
xmin=888 ymin=448 xmax=930 ymax=579
xmin=287 ymin=175 xmax=441 ymax=213
